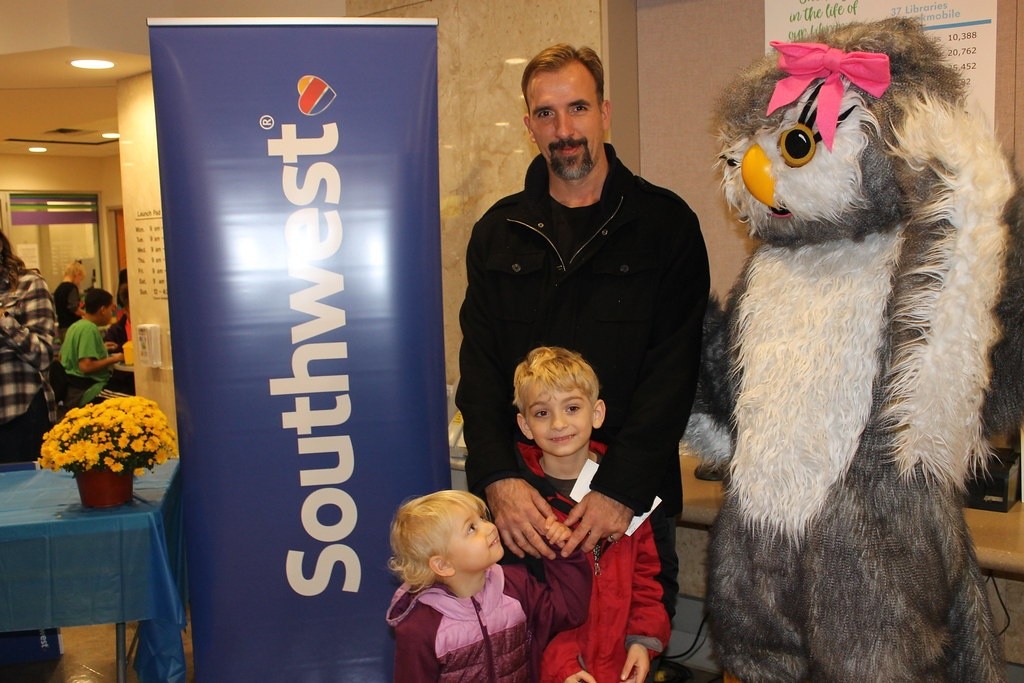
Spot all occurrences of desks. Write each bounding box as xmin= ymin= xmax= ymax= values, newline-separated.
xmin=0 ymin=458 xmax=183 ymax=683
xmin=114 ymin=363 xmax=134 ymax=372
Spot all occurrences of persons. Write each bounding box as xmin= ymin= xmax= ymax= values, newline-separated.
xmin=0 ymin=231 xmax=133 ymax=466
xmin=455 ymin=43 xmax=711 ymax=683
xmin=385 ymin=490 xmax=590 ymax=683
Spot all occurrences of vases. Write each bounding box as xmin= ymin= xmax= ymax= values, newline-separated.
xmin=72 ymin=465 xmax=134 ymax=510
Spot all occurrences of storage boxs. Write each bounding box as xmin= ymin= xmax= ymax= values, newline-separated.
xmin=122 ymin=340 xmax=136 ymax=365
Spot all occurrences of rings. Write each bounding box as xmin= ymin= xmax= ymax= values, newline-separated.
xmin=610 ymin=535 xmax=615 ymax=541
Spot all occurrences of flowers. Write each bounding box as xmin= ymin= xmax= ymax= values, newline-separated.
xmin=37 ymin=394 xmax=180 ymax=476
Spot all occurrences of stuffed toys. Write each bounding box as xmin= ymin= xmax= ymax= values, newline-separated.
xmin=678 ymin=16 xmax=1024 ymax=683
